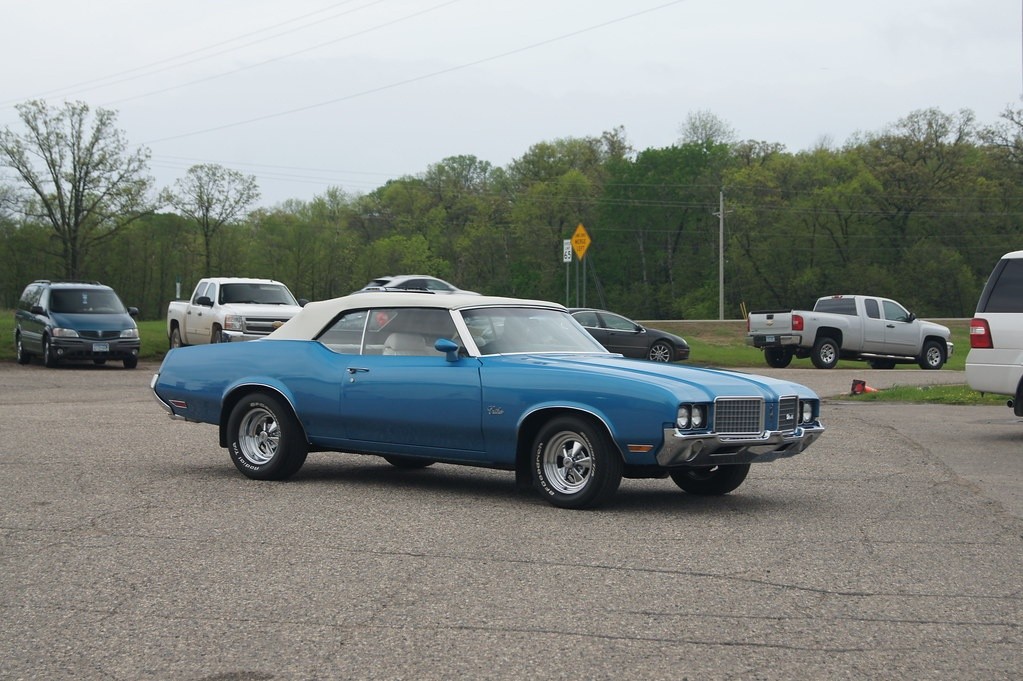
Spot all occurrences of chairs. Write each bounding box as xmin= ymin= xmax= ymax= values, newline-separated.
xmin=453 ymin=336 xmax=486 ymax=347
xmin=384 ymin=333 xmax=429 ymax=356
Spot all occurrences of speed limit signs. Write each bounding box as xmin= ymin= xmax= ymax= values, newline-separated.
xmin=563 ymin=239 xmax=572 ymax=263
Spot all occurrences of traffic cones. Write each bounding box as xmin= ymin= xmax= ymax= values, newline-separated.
xmin=850 ymin=379 xmax=879 ymax=395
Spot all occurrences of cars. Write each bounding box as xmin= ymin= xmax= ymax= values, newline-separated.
xmin=148 ymin=289 xmax=826 ymax=511
xmin=321 ymin=273 xmax=690 ymax=364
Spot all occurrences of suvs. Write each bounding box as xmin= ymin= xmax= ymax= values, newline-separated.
xmin=966 ymin=251 xmax=1023 ymax=418
xmin=15 ymin=280 xmax=141 ymax=370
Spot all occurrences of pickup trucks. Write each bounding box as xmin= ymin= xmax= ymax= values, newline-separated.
xmin=748 ymin=295 xmax=954 ymax=371
xmin=167 ymin=276 xmax=309 ymax=351
